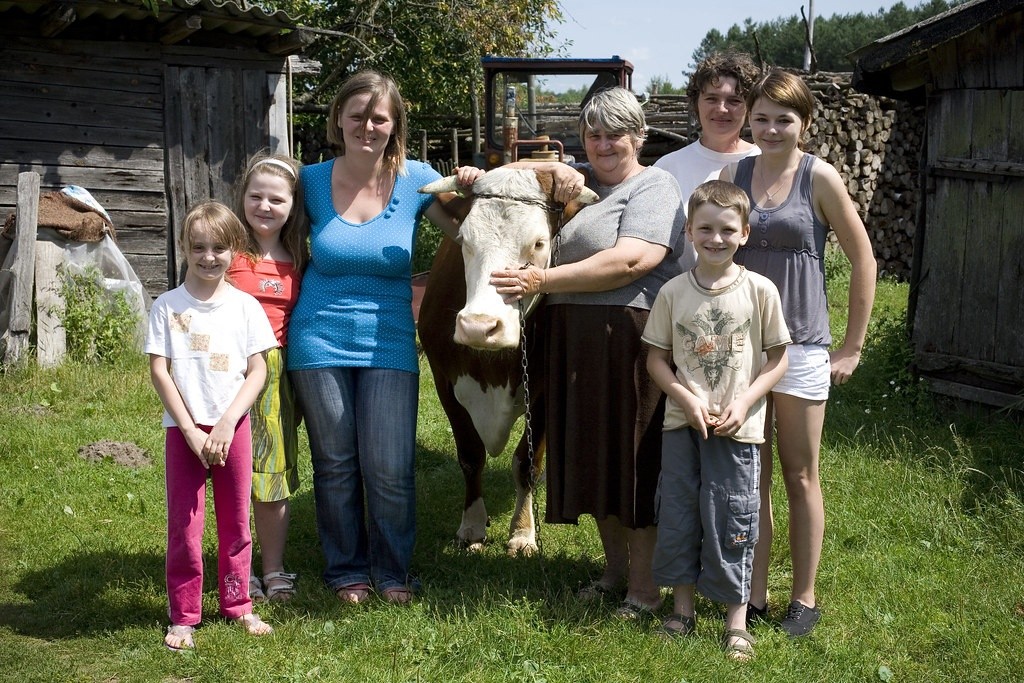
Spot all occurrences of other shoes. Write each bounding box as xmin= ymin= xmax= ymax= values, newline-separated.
xmin=578 ymin=585 xmax=612 ymax=609
xmin=337 ymin=583 xmax=368 ymax=605
xmin=614 ymin=601 xmax=655 ymax=630
xmin=381 ymin=588 xmax=412 ymax=604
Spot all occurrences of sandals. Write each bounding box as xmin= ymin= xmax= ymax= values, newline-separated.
xmin=664 ymin=614 xmax=696 ymax=644
xmin=263 ymin=572 xmax=297 ymax=602
xmin=248 ymin=576 xmax=264 ymax=604
xmin=723 ymin=630 xmax=756 ymax=664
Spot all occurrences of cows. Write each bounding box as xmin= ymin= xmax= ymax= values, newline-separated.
xmin=417 ymin=159 xmax=595 ymax=553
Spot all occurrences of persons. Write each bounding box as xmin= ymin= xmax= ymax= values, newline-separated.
xmin=489 ymin=86 xmax=687 ymax=631
xmin=652 ymin=51 xmax=761 ymax=272
xmin=638 ymin=179 xmax=792 ymax=662
xmin=717 ymin=70 xmax=878 ymax=638
xmin=225 ymin=153 xmax=307 ymax=608
xmin=143 ymin=201 xmax=279 ymax=652
xmin=298 ymin=70 xmax=485 ymax=604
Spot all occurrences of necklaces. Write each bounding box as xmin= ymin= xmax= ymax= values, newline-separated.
xmin=760 ymin=171 xmax=789 ymax=201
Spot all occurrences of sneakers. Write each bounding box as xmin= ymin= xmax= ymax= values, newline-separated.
xmin=774 ymin=600 xmax=818 ymax=638
xmin=745 ymin=602 xmax=768 ymax=629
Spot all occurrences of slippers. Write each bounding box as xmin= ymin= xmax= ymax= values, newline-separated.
xmin=164 ymin=625 xmax=194 ymax=652
xmin=237 ymin=614 xmax=273 ymax=636
